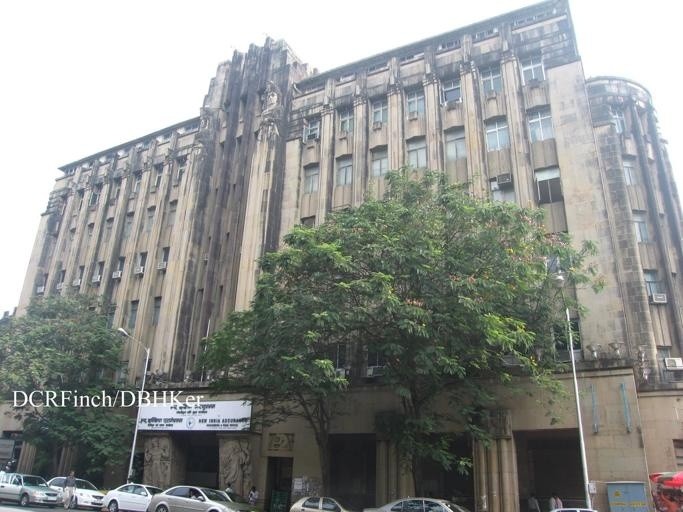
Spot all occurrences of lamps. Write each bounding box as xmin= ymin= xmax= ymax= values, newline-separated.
xmin=586 ymin=339 xmax=623 ymax=363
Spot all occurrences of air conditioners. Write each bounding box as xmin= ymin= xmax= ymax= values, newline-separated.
xmin=650 ymin=293 xmax=668 ymax=304
xmin=333 ymin=364 xmax=390 ymax=380
xmin=35 ymin=260 xmax=167 ymax=294
xmin=663 ymin=357 xmax=683 ymax=372
xmin=496 ymin=172 xmax=513 ymax=190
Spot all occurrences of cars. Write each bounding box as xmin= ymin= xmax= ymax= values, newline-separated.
xmin=1 ymin=473 xmax=105 ymax=509
xmin=102 ymin=482 xmax=255 ymax=512
xmin=290 ymin=496 xmax=357 ymax=512
xmin=363 ymin=497 xmax=472 ymax=512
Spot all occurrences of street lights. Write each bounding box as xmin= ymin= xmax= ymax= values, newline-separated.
xmin=555 ymin=276 xmax=594 ymax=509
xmin=118 ymin=327 xmax=150 ymax=483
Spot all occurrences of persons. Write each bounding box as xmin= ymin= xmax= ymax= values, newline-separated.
xmin=547 ymin=494 xmax=556 ymax=512
xmin=554 ymin=494 xmax=563 ymax=510
xmin=526 ymin=493 xmax=540 ymax=512
xmin=655 ymin=489 xmax=683 ymax=512
xmin=61 ymin=470 xmax=77 ymax=510
xmin=247 ymin=486 xmax=259 ymax=507
xmin=223 ymin=482 xmax=234 ymax=498
